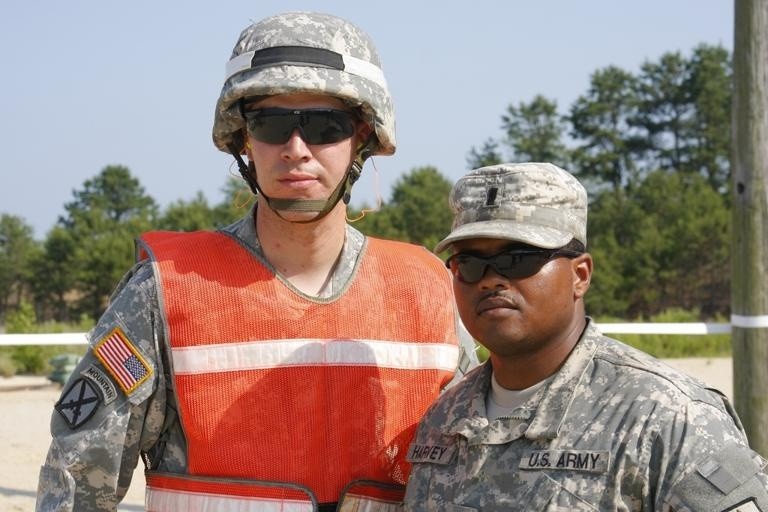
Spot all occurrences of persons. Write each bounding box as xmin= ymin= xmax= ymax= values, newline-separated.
xmin=27 ymin=7 xmax=483 ymax=511
xmin=400 ymin=159 xmax=768 ymax=511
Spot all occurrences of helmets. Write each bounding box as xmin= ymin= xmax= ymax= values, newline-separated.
xmin=211 ymin=11 xmax=396 ymax=157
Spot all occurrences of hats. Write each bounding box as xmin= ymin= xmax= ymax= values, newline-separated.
xmin=435 ymin=161 xmax=589 ymax=255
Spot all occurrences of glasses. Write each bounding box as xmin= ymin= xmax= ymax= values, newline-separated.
xmin=446 ymin=242 xmax=584 ymax=284
xmin=242 ymin=106 xmax=368 ymax=147
